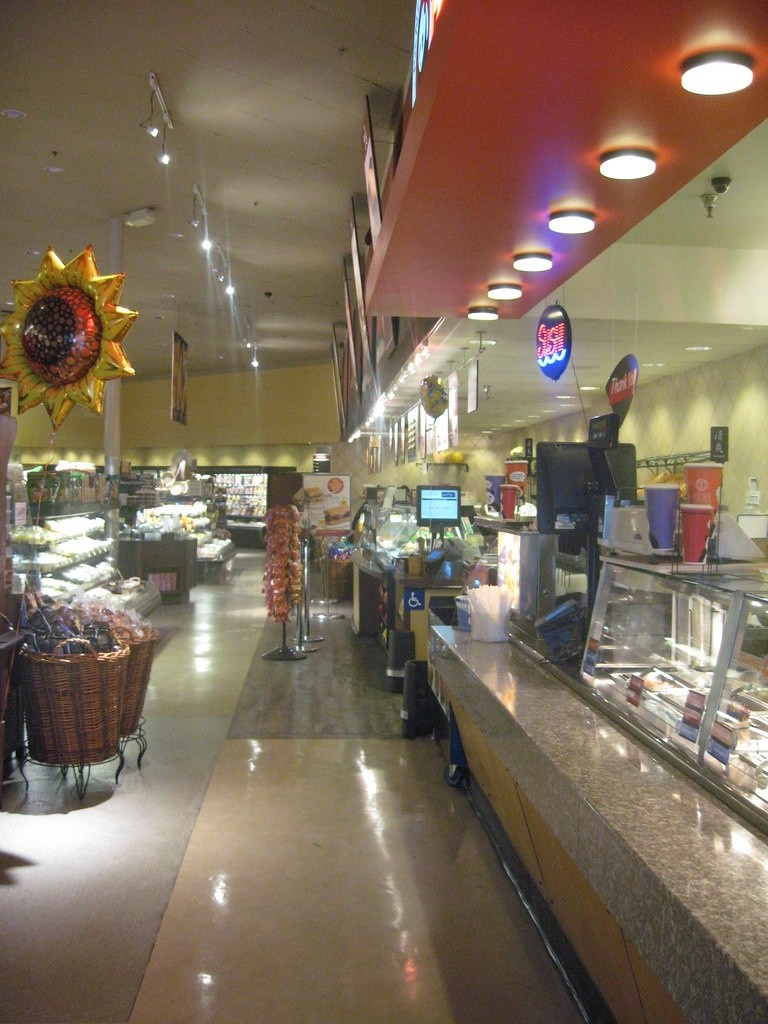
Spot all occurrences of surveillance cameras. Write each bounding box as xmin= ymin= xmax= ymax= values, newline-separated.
xmin=265 ymin=293 xmax=272 ymax=297
xmin=711 ymin=176 xmax=731 ymax=194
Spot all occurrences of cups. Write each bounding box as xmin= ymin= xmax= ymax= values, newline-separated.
xmin=679 ymin=504 xmax=717 ymax=565
xmin=643 ymin=483 xmax=680 ymax=552
xmin=504 ymin=460 xmax=529 ymax=499
xmin=498 ymin=484 xmax=519 ymax=522
xmin=484 ymin=473 xmax=507 ymax=512
xmin=682 ymin=462 xmax=724 ymax=513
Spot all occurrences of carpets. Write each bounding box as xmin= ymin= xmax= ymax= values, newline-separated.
xmin=224 ymin=610 xmax=401 ymax=739
xmin=0 ymin=715 xmax=226 ymax=1024
xmin=152 ymin=625 xmax=180 ymax=649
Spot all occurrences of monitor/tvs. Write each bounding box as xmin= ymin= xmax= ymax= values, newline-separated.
xmin=416 ymin=484 xmax=461 ymax=527
xmin=535 ymin=440 xmax=637 ymax=535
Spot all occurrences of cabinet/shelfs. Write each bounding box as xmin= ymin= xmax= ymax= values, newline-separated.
xmin=213 ymin=472 xmax=270 ymax=520
xmin=138 ymin=455 xmax=239 ymax=584
xmin=11 ymin=509 xmax=119 ymax=604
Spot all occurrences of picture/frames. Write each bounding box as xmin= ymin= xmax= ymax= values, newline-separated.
xmin=302 ymin=473 xmax=351 ymax=530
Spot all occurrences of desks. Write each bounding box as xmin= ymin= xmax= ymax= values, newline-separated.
xmin=118 ymin=537 xmax=198 ymax=606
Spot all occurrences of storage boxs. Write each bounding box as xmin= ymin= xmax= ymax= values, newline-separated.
xmin=610 ymin=669 xmax=768 ymax=803
xmin=120 ymin=459 xmax=138 ymax=480
xmin=27 ymin=469 xmax=95 ymax=503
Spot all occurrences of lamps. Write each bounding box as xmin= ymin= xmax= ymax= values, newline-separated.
xmin=488 ymin=284 xmax=522 ymax=301
xmin=512 ymin=253 xmax=552 ymax=272
xmin=189 ymin=195 xmax=199 ymax=227
xmin=548 ymin=211 xmax=595 ymax=235
xmin=155 ymin=123 xmax=170 ymax=165
xmin=137 ymin=89 xmax=159 ymax=138
xmin=242 ymin=325 xmax=259 ymax=368
xmin=216 ymin=253 xmax=225 ymax=282
xmin=680 ymin=52 xmax=754 ymax=96
xmin=467 ymin=307 xmax=499 ymax=321
xmin=598 ymin=148 xmax=657 ymax=180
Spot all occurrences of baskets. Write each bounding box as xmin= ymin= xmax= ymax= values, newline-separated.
xmin=121 ymin=626 xmax=159 ymax=735
xmin=17 ymin=638 xmax=131 ymax=765
